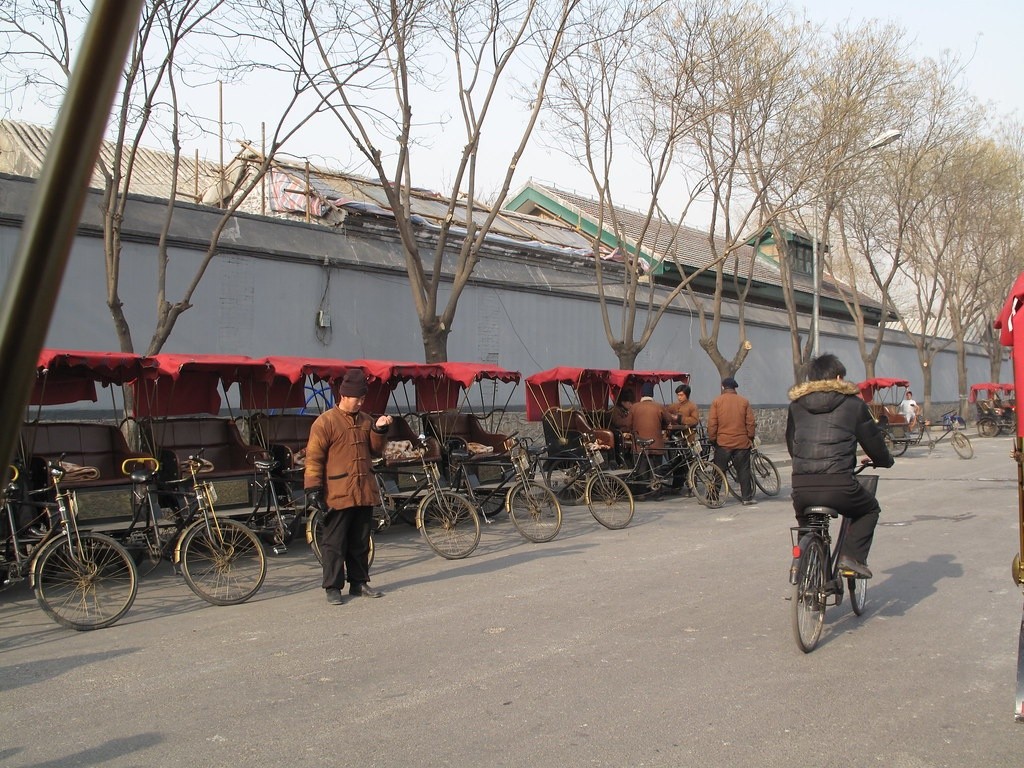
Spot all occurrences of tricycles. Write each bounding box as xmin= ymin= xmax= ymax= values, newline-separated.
xmin=854 ymin=376 xmax=974 ymax=460
xmin=348 ymin=360 xmax=483 ymax=559
xmin=524 ymin=364 xmax=730 ymax=509
xmin=390 ymin=363 xmax=562 ymax=542
xmin=0 ymin=462 xmax=141 ymax=632
xmin=128 ymin=353 xmax=375 ymax=579
xmin=968 ymin=382 xmax=1016 ymax=438
xmin=11 ymin=348 xmax=268 ymax=606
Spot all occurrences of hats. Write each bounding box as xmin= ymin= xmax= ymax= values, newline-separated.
xmin=722 ymin=378 xmax=738 ymax=386
xmin=339 ymin=368 xmax=369 ymax=398
xmin=641 ymin=383 xmax=653 ymax=397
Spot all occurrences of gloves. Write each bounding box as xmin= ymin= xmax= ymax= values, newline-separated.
xmin=306 ymin=490 xmax=327 ymax=511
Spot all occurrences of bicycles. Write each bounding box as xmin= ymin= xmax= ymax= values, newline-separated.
xmin=783 ymin=459 xmax=880 ymax=653
xmin=725 ymin=435 xmax=781 ymax=502
xmin=526 ymin=438 xmax=635 ymax=531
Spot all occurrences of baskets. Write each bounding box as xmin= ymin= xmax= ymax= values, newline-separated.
xmin=856 ymin=475 xmax=879 ymax=496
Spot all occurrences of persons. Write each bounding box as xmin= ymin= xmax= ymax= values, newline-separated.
xmin=665 ymin=384 xmax=699 ymax=496
xmin=784 ymin=354 xmax=895 ymax=606
xmin=627 ymin=382 xmax=672 ymax=501
xmin=607 ymin=389 xmax=635 ymax=433
xmin=900 ymin=391 xmax=916 ymax=422
xmin=304 ymin=368 xmax=393 ymax=604
xmin=698 ymin=378 xmax=757 ymax=505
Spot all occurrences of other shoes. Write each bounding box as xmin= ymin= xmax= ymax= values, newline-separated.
xmin=742 ymin=498 xmax=758 ymax=505
xmin=636 ymin=497 xmax=645 ymax=501
xmin=837 ymin=556 xmax=873 ymax=579
xmin=662 ymin=488 xmax=682 ymax=495
xmin=804 ymin=598 xmax=819 ymax=612
xmin=653 ymin=494 xmax=664 ymax=500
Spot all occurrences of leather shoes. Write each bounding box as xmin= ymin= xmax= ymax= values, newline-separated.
xmin=325 ymin=586 xmax=343 ymax=605
xmin=349 ymin=582 xmax=382 ymax=598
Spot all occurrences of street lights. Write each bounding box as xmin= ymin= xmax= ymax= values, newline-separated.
xmin=812 ymin=130 xmax=902 ymax=361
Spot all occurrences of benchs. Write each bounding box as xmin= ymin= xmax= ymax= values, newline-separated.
xmin=254 ymin=415 xmax=322 ymax=479
xmin=15 ymin=419 xmax=156 ymax=494
xmin=373 ymin=416 xmax=441 ymax=464
xmin=980 ymin=399 xmax=1009 ymax=416
xmin=143 ymin=418 xmax=270 ymax=481
xmin=544 ymin=410 xmax=614 ymax=451
xmin=422 ymin=412 xmax=510 ymax=463
xmin=869 ymin=403 xmax=906 ymax=424
